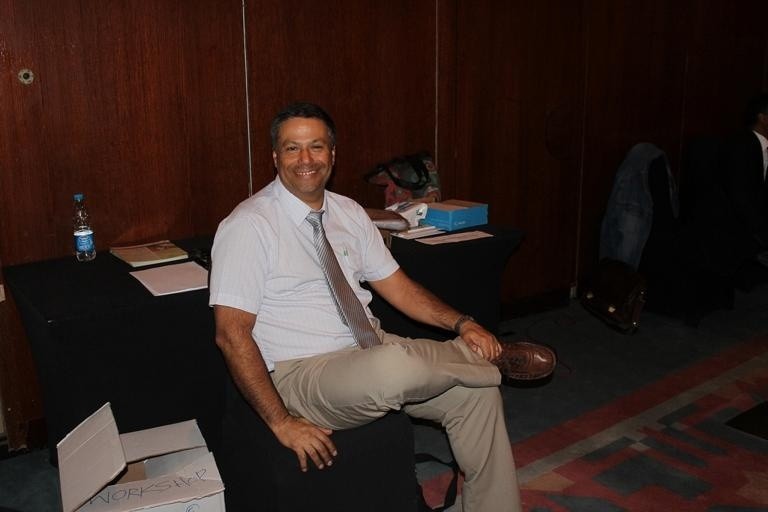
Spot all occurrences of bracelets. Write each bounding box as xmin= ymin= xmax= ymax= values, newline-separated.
xmin=452 ymin=314 xmax=476 ymax=338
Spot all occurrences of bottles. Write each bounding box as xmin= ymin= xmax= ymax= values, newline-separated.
xmin=71 ymin=193 xmax=97 ymax=264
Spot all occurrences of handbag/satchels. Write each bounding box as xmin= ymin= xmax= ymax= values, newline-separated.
xmin=579 ymin=256 xmax=647 ymax=336
xmin=355 ymin=143 xmax=441 ymax=209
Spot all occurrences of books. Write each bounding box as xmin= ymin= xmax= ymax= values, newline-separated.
xmin=106 ymin=238 xmax=190 ymax=268
xmin=128 ymin=259 xmax=210 ymax=298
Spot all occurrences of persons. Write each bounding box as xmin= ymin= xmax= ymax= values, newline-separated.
xmin=206 ymin=101 xmax=558 ymax=512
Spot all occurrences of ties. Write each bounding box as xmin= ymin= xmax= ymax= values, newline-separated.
xmin=305 ymin=210 xmax=383 ymax=350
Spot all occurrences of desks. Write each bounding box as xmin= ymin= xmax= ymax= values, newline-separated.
xmin=4 ymin=217 xmax=527 ymax=469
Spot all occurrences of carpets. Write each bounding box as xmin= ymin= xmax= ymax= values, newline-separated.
xmin=419 ymin=336 xmax=768 ymax=512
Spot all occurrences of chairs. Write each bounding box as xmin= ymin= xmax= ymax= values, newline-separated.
xmin=222 ymin=368 xmax=419 ymax=512
xmin=633 ymin=146 xmax=735 ymax=327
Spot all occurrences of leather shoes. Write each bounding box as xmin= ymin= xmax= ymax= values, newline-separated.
xmin=491 ymin=340 xmax=558 ymax=381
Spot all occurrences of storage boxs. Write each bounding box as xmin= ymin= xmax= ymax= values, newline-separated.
xmin=56 ymin=402 xmax=227 ymax=512
